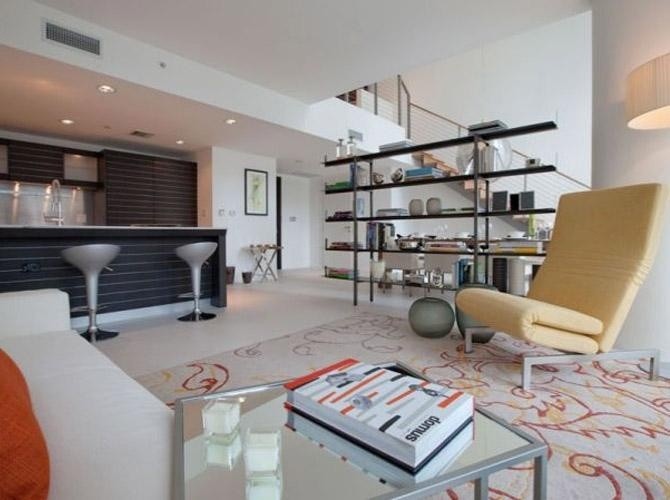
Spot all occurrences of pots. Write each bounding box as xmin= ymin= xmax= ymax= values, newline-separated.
xmin=394 ymin=233 xmax=425 ymax=250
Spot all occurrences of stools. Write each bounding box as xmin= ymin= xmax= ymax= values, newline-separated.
xmin=173 ymin=241 xmax=220 ymax=323
xmin=59 ymin=244 xmax=121 ymax=342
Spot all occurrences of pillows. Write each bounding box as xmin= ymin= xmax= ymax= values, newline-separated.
xmin=0 ymin=349 xmax=50 ymax=498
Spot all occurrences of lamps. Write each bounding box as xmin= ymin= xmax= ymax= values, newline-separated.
xmin=623 ymin=55 xmax=670 ymax=382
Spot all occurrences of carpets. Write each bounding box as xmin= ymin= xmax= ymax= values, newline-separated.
xmin=131 ymin=311 xmax=670 ymax=500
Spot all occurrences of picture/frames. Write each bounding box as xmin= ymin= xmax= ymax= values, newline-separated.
xmin=244 ymin=168 xmax=269 ymax=216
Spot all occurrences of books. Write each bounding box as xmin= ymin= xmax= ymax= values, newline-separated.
xmin=283 ymin=357 xmax=475 ymax=492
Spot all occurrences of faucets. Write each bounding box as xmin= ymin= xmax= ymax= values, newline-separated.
xmin=44 ymin=178 xmax=64 ymax=225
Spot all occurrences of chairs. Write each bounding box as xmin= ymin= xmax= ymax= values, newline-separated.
xmin=381 ymin=246 xmax=469 ymax=295
xmin=456 ymin=181 xmax=666 ymax=395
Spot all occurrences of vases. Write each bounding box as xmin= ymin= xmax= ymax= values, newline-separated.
xmin=409 ymin=282 xmax=501 ymax=344
xmin=409 ymin=198 xmax=441 ymax=216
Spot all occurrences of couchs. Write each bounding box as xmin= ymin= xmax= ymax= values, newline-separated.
xmin=0 ymin=287 xmax=174 ymax=500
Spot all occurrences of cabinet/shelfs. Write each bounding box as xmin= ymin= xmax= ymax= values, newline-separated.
xmin=322 ymin=120 xmax=560 ymax=307
